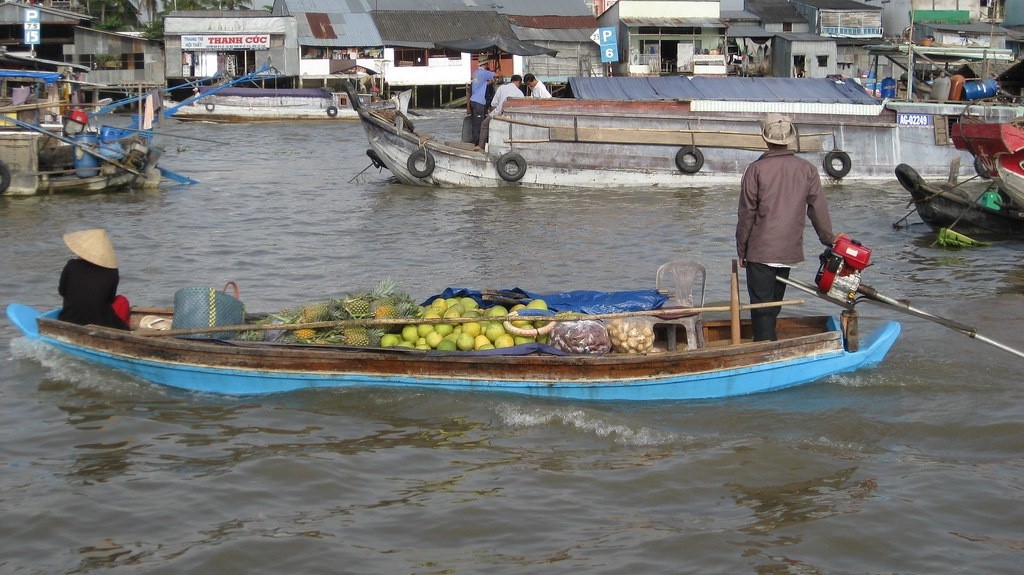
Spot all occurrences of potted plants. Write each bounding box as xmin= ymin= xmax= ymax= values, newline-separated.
xmin=919 ymin=38 xmax=933 ymax=46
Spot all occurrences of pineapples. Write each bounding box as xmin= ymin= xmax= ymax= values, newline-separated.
xmin=281 ymin=276 xmax=423 ymax=348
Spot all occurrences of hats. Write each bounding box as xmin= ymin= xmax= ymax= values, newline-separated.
xmin=759 ymin=113 xmax=796 ymax=145
xmin=63 ymin=228 xmax=118 ymax=269
xmin=477 ymin=56 xmax=488 ymax=65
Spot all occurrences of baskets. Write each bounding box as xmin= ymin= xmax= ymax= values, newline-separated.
xmin=170 ymin=281 xmax=245 ymax=341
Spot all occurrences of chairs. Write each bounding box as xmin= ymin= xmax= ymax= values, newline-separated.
xmin=655 ymin=261 xmax=706 ymax=351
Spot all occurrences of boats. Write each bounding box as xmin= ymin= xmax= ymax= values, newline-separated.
xmin=6 ymin=234 xmax=900 ymax=400
xmin=161 ymin=70 xmax=412 ymax=123
xmin=0 ymin=46 xmax=165 ymax=195
xmin=343 ymin=8 xmax=1024 ymax=249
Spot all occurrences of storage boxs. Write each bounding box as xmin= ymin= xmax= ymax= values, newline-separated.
xmin=64 ymin=119 xmax=83 ymax=134
xmin=70 ymin=110 xmax=87 ymax=123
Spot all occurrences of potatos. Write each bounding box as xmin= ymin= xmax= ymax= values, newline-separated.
xmin=548 ymin=319 xmax=612 ymax=354
xmin=605 ymin=317 xmax=660 ymax=355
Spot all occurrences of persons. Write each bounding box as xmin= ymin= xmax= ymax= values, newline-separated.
xmin=470 ymin=55 xmax=503 ymax=147
xmin=479 ymin=75 xmax=525 ymax=149
xmin=735 ymin=113 xmax=844 ymax=342
xmin=58 ymin=229 xmax=131 ymax=331
xmin=523 ymin=74 xmax=552 ymax=98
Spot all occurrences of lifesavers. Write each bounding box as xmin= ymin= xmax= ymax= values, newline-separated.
xmin=407 ymin=150 xmax=435 ymax=178
xmin=974 ymin=157 xmax=991 ymax=180
xmin=206 ymin=103 xmax=214 ymax=112
xmin=676 ymin=146 xmax=704 ymax=173
xmin=497 ymin=152 xmax=527 ymax=182
xmin=823 ymin=149 xmax=852 ymax=179
xmin=366 ymin=148 xmax=388 ymax=169
xmin=502 ymin=308 xmax=558 ymax=337
xmin=326 ymin=105 xmax=337 ymax=117
xmin=895 ymin=163 xmax=921 ymax=193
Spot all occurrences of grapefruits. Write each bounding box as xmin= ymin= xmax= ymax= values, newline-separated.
xmin=382 ymin=297 xmax=550 ymax=353
